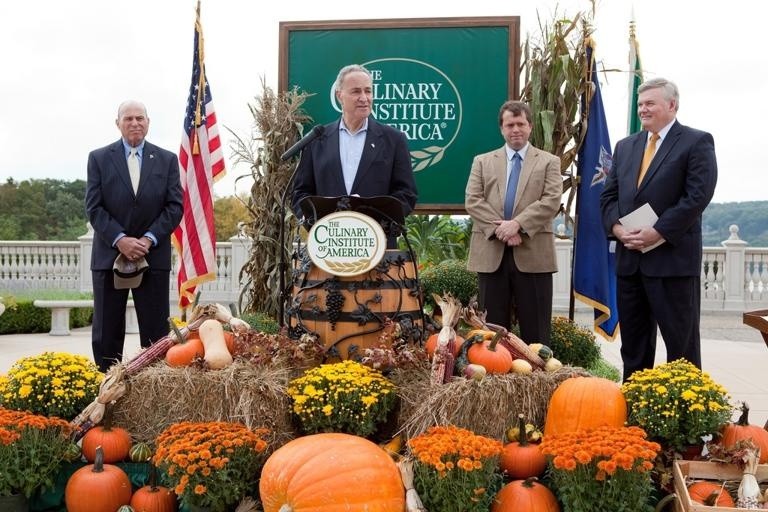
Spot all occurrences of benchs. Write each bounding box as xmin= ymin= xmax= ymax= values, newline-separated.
xmin=31 ymin=295 xmax=141 ymax=338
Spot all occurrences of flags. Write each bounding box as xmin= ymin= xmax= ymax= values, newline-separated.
xmin=174 ymin=19 xmax=225 ymax=314
xmin=623 ymin=46 xmax=646 ymax=138
xmin=572 ymin=38 xmax=625 ymax=341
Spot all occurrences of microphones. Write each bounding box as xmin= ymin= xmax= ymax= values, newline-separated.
xmin=280 ymin=124 xmax=325 ymax=160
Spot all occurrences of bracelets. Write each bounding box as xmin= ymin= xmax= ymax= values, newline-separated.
xmin=146 ymin=236 xmax=154 ymax=242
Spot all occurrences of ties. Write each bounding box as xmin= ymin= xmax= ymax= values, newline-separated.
xmin=504 ymin=153 xmax=522 ymax=220
xmin=638 ymin=134 xmax=660 ymax=189
xmin=127 ymin=148 xmax=140 ymax=195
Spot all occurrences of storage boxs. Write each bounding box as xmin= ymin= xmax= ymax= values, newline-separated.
xmin=670 ymin=457 xmax=768 ymax=512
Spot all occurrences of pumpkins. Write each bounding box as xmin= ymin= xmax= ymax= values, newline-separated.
xmin=58 ymin=398 xmax=181 ymax=511
xmin=685 ymin=478 xmax=735 ymax=509
xmin=718 ymin=400 xmax=768 ymax=465
xmin=257 ymin=433 xmax=407 ymax=512
xmin=422 ymin=325 xmax=563 ymax=381
xmin=545 ymin=376 xmax=627 ymax=431
xmin=165 ymin=313 xmax=236 ymax=369
xmin=488 ymin=475 xmax=561 ymax=511
xmin=499 ymin=412 xmax=546 ymax=478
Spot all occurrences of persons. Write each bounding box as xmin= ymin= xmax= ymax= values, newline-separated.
xmin=462 ymin=100 xmax=563 ymax=347
xmin=87 ymin=99 xmax=185 ymax=370
xmin=291 ymin=64 xmax=420 ymax=251
xmin=600 ymin=77 xmax=717 ymax=387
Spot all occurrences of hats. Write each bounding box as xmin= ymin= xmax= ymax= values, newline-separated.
xmin=112 ymin=252 xmax=149 ymax=290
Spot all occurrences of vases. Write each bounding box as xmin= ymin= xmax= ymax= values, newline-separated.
xmin=0 ymin=492 xmax=23 ymax=512
xmin=657 ymin=431 xmax=706 ymax=463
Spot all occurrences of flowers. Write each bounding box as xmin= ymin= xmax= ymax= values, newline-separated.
xmin=287 ymin=359 xmax=395 ymax=438
xmin=405 ymin=424 xmax=506 ymax=512
xmin=0 ymin=348 xmax=107 ymax=417
xmin=621 ymin=355 xmax=734 ymax=444
xmin=536 ymin=422 xmax=663 ymax=512
xmin=0 ymin=410 xmax=83 ymax=499
xmin=150 ymin=418 xmax=271 ymax=511
